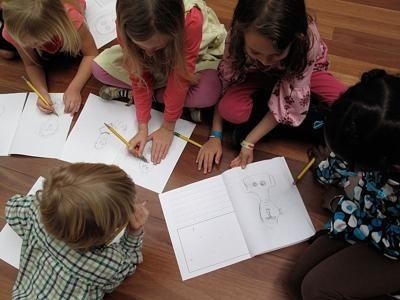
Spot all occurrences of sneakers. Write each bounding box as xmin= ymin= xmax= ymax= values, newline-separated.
xmin=100 ymin=86 xmax=132 ymax=100
xmin=190 ymin=108 xmax=202 ymax=122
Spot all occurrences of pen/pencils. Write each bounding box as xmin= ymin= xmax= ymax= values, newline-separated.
xmin=20 ymin=75 xmax=58 ymax=116
xmin=292 ymin=156 xmax=316 ymax=186
xmin=104 ymin=123 xmax=150 ymax=164
xmin=173 ymin=132 xmax=203 ymax=149
xmin=99 ymin=200 xmax=148 ymax=251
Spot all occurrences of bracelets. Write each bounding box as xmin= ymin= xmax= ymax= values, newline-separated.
xmin=240 ymin=140 xmax=254 ymax=150
xmin=209 ymin=130 xmax=223 ymax=140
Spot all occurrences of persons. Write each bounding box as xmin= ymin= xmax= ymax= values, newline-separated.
xmin=4 ymin=161 xmax=150 ymax=300
xmin=196 ymin=0 xmax=348 ymax=174
xmin=91 ymin=0 xmax=228 ymax=166
xmin=290 ymin=69 xmax=400 ymax=300
xmin=0 ymin=0 xmax=98 ymax=113
